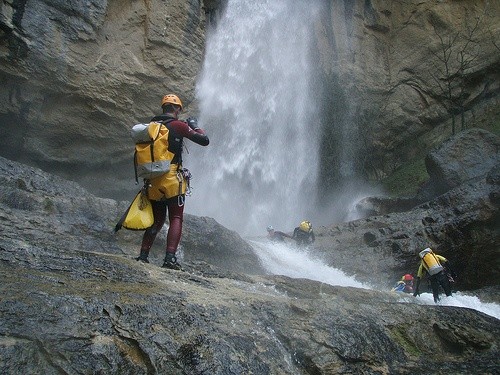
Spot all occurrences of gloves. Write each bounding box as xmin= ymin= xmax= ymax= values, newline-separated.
xmin=445 ymin=260 xmax=456 ymax=272
xmin=413 ymin=277 xmax=421 ymax=296
xmin=186 ymin=116 xmax=200 ymax=131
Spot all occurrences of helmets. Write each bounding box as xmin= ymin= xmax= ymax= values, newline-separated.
xmin=161 ymin=94 xmax=184 ymax=109
xmin=266 ymin=225 xmax=274 ymax=231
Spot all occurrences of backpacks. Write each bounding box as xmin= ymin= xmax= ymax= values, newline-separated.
xmin=133 ymin=118 xmax=185 ymax=184
xmin=300 ymin=221 xmax=312 ymax=232
xmin=419 ymin=247 xmax=443 ymax=275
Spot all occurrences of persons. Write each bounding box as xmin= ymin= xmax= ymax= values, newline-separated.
xmin=266 ymin=225 xmax=301 ymax=246
xmin=293 ymin=221 xmax=315 ymax=249
xmin=393 ymin=271 xmax=415 ymax=292
xmin=412 ymin=248 xmax=452 ymax=304
xmin=137 ymin=93 xmax=210 ymax=273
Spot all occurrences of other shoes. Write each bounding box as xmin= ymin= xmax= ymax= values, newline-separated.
xmin=162 ymin=252 xmax=182 ymax=270
xmin=136 ymin=250 xmax=150 ymax=263
